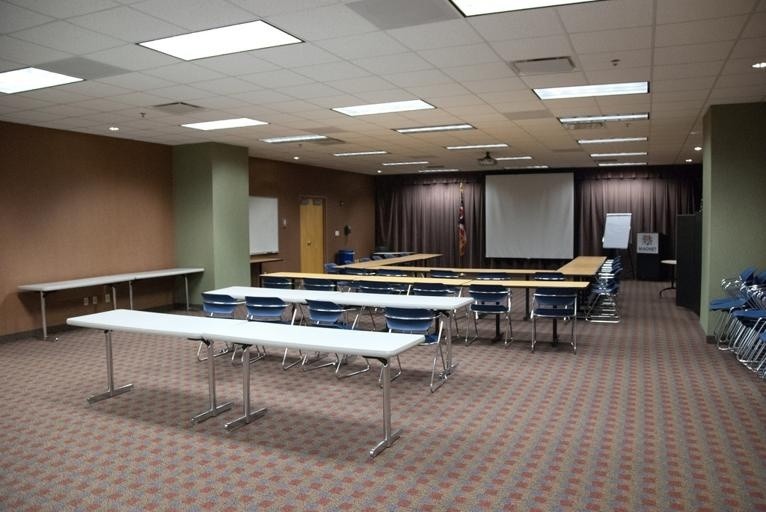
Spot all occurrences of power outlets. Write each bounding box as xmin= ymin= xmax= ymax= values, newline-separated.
xmin=83 ymin=294 xmax=111 ymax=307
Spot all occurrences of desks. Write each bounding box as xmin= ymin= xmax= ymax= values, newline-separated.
xmin=113 ymin=267 xmax=206 ymax=313
xmin=658 ymin=259 xmax=676 ymax=297
xmin=200 ymin=318 xmax=428 ymax=461
xmin=64 ymin=306 xmax=248 ymax=426
xmin=18 ymin=275 xmax=134 ymax=343
xmin=201 ymin=286 xmax=475 ymax=379
xmin=557 ymin=255 xmax=607 ymax=305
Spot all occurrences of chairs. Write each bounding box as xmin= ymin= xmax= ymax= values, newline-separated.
xmin=378 ymin=307 xmax=448 ymax=391
xmin=241 ymin=295 xmax=303 ymax=371
xmin=584 ymin=255 xmax=623 ymax=324
xmin=257 ymin=254 xmax=557 ymax=341
xmin=529 ymin=287 xmax=578 ymax=354
xmin=466 ymin=284 xmax=514 ymax=347
xmin=532 ymin=272 xmax=567 ymax=281
xmin=302 ymin=299 xmax=370 ymax=379
xmin=197 ymin=292 xmax=261 ymax=366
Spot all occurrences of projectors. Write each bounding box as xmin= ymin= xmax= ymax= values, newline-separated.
xmin=478 ymin=158 xmax=496 ymax=165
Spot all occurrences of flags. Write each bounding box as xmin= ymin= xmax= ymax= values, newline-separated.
xmin=456 ymin=182 xmax=469 ymax=260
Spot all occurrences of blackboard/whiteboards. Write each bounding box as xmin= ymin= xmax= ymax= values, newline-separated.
xmin=249 ymin=196 xmax=279 ymax=256
xmin=602 ymin=213 xmax=632 ymax=249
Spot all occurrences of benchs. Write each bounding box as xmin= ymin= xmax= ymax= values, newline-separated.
xmin=708 ymin=265 xmax=766 ymax=383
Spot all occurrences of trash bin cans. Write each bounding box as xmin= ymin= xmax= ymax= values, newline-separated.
xmin=337 ymin=250 xmax=355 ymax=265
